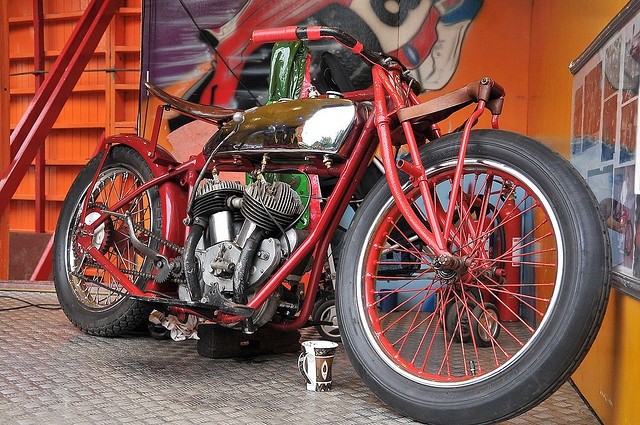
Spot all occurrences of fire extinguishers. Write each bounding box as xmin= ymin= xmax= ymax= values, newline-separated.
xmin=462 ymin=194 xmax=505 ymax=305
xmin=494 ymin=180 xmax=522 ymax=322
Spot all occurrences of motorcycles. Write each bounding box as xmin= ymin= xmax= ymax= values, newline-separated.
xmin=52 ymin=23 xmax=614 ymax=425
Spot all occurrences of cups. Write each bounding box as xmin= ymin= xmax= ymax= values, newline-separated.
xmin=297 ymin=339 xmax=339 ymax=393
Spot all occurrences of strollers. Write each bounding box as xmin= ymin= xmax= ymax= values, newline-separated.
xmin=245 ymin=51 xmax=502 ymax=348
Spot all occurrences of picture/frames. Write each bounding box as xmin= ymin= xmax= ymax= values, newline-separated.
xmin=567 ymin=0 xmax=640 ymax=300
